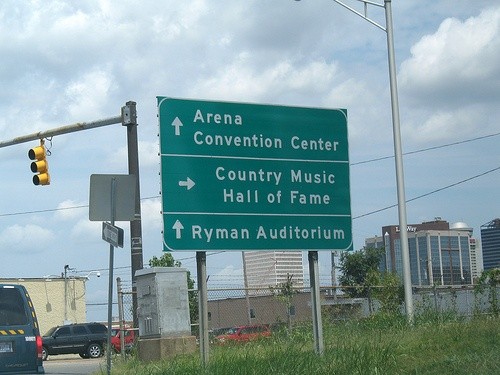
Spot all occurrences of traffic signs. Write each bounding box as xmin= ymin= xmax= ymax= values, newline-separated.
xmin=155 ymin=96 xmax=355 ymax=252
xmin=102 ymin=221 xmax=119 ymax=248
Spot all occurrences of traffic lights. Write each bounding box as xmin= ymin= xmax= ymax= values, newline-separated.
xmin=28 ymin=144 xmax=51 ymax=186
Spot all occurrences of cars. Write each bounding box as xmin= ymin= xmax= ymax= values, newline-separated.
xmin=106 ymin=324 xmax=135 ymax=354
xmin=210 ymin=324 xmax=271 ymax=346
xmin=195 ymin=327 xmax=230 ymax=345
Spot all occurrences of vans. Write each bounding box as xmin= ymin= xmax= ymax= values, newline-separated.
xmin=0 ymin=284 xmax=45 ymax=374
xmin=42 ymin=322 xmax=108 ymax=361
